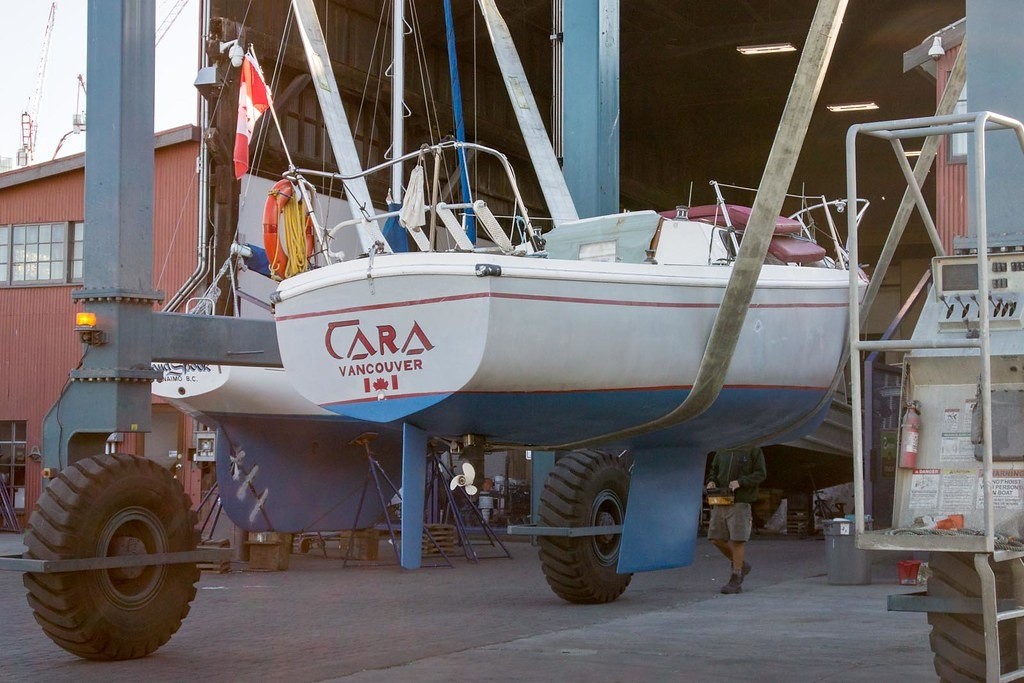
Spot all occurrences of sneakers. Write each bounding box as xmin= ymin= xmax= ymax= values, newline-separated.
xmin=721 ymin=561 xmax=751 ymax=594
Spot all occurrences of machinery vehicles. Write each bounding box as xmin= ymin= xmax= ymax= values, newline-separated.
xmin=841 ymin=0 xmax=1024 ymax=683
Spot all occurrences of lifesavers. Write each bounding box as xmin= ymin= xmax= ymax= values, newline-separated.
xmin=263 ymin=179 xmax=315 ymax=281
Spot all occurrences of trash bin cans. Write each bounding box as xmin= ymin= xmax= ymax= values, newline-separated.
xmin=817 ymin=512 xmax=876 ymax=588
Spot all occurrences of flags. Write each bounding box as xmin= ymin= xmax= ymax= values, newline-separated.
xmin=233 ymin=53 xmax=272 ymax=181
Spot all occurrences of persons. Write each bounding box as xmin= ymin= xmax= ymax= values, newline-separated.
xmin=706 ymin=445 xmax=767 ymax=594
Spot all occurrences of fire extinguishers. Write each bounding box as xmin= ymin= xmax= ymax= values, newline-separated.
xmin=899 ymin=399 xmax=922 ymax=470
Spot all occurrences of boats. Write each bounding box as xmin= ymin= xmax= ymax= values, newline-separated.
xmin=147 ymin=0 xmax=870 ymax=574
